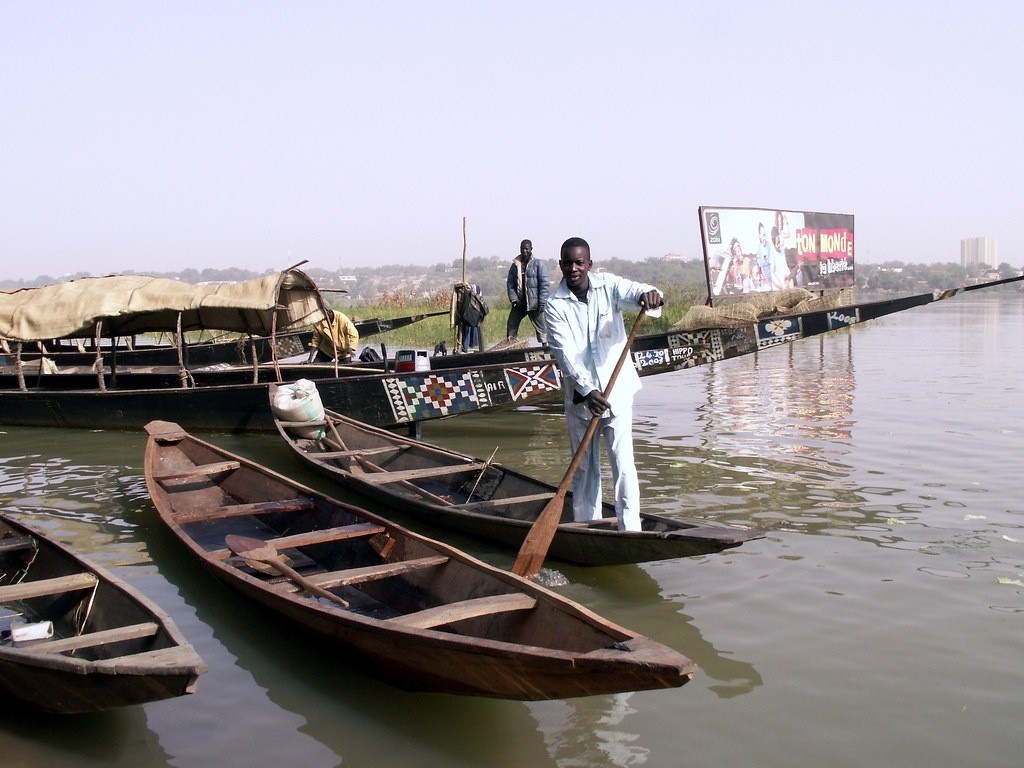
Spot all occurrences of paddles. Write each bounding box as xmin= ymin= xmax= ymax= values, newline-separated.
xmin=325 ymin=414 xmax=365 ymax=474
xmin=511 ymin=298 xmax=663 ymax=577
xmin=225 ymin=534 xmax=350 ymax=608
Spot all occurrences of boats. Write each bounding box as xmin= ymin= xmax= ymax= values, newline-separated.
xmin=2 ymin=511 xmax=205 ymax=724
xmin=143 ymin=417 xmax=698 ymax=701
xmin=3 ymin=309 xmax=449 ymax=364
xmin=1 ymin=263 xmax=1024 ymax=427
xmin=270 ymin=384 xmax=758 ymax=568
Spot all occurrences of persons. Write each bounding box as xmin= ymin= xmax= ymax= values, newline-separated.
xmin=766 ymin=227 xmax=794 ymax=288
xmin=730 ymin=238 xmax=750 ymax=288
xmin=543 ymin=238 xmax=662 ymax=533
xmin=506 ymin=239 xmax=549 ymax=348
xmin=775 ymin=212 xmax=785 ymax=229
xmin=757 ymin=224 xmax=771 ymax=274
xmin=302 ymin=297 xmax=359 ymax=366
xmin=744 ymin=261 xmax=769 ymax=291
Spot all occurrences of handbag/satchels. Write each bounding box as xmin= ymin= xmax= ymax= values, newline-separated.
xmin=462 ymin=291 xmax=480 ymax=329
